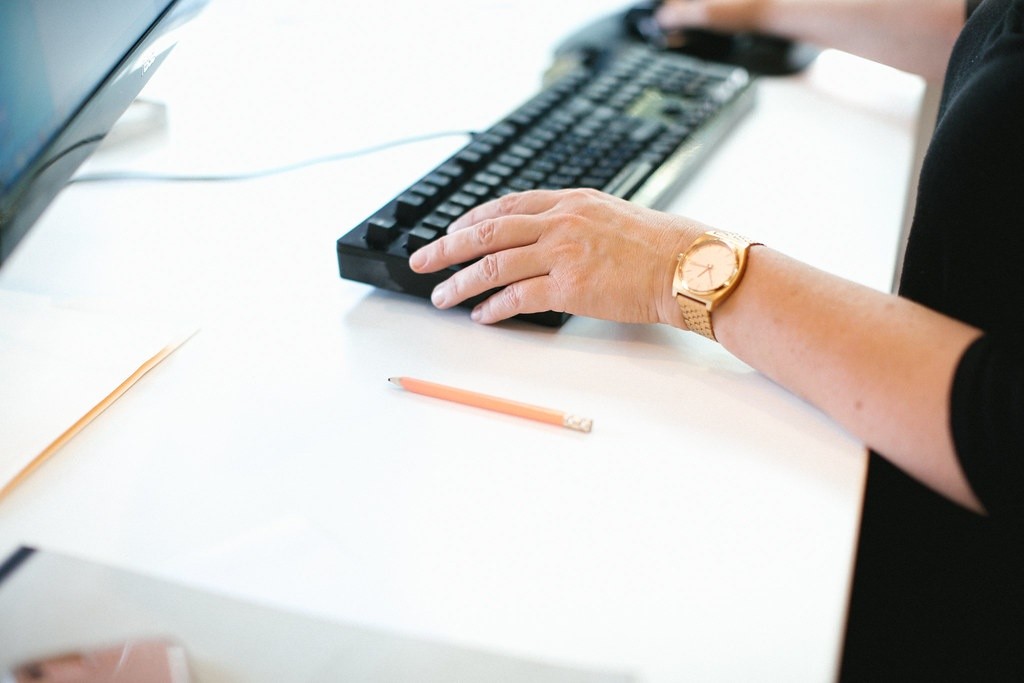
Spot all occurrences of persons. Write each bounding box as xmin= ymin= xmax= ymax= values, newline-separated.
xmin=409 ymin=1 xmax=1024 ymax=683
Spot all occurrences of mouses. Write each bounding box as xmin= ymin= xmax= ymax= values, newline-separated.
xmin=622 ymin=0 xmax=817 ymax=77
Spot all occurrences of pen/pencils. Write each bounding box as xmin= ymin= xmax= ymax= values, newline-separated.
xmin=386 ymin=374 xmax=592 ymax=436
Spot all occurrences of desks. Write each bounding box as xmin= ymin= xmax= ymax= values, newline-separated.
xmin=0 ymin=0 xmax=925 ymax=683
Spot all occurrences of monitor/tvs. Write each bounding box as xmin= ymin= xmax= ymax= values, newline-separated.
xmin=0 ymin=0 xmax=210 ymax=264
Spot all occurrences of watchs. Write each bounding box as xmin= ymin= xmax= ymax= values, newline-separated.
xmin=672 ymin=230 xmax=766 ymax=343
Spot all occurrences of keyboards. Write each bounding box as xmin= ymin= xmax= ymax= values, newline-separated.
xmin=337 ymin=40 xmax=757 ymax=328
